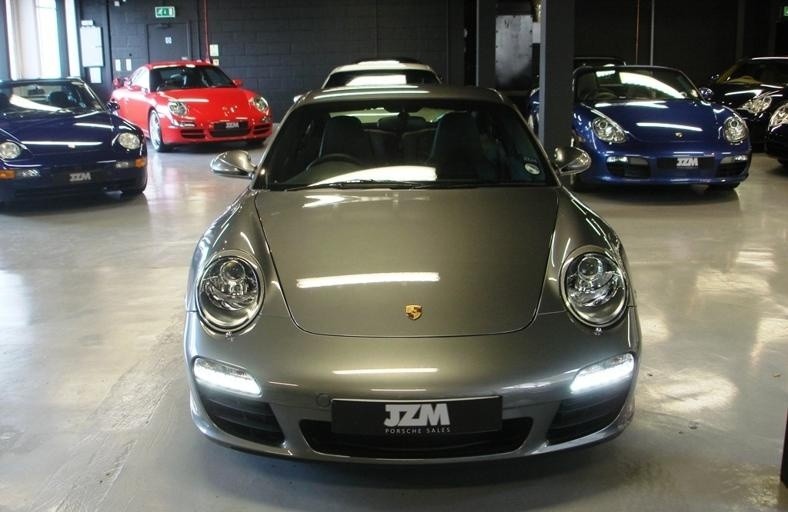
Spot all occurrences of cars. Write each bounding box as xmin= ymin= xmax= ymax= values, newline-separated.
xmin=527 ymin=56 xmax=788 ymax=196
xmin=110 ymin=60 xmax=274 ymax=154
xmin=2 ymin=77 xmax=148 ymax=209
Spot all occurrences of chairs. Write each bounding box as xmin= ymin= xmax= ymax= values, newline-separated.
xmin=628 ymin=84 xmax=650 ymax=98
xmin=303 ymin=108 xmax=498 ymax=170
xmin=47 ymin=90 xmax=73 ymax=108
